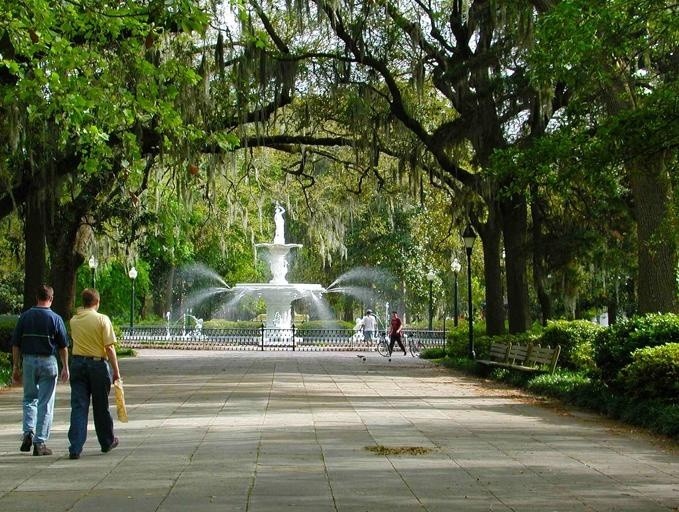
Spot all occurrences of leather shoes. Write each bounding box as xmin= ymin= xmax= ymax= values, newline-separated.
xmin=20 ymin=435 xmax=33 ymax=452
xmin=101 ymin=438 xmax=120 ymax=453
xmin=33 ymin=442 xmax=52 ymax=456
xmin=69 ymin=453 xmax=81 ymax=459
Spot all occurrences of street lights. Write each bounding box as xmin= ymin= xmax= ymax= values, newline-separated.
xmin=127 ymin=267 xmax=137 ymax=335
xmin=186 ymin=277 xmax=194 ymax=325
xmin=461 ymin=227 xmax=478 ymax=359
xmin=426 ymin=270 xmax=435 ymax=330
xmin=449 ymin=258 xmax=461 ymax=327
xmin=87 ymin=255 xmax=98 ymax=289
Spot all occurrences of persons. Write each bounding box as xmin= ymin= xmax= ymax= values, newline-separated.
xmin=12 ymin=284 xmax=70 ymax=456
xmin=68 ymin=288 xmax=120 ymax=459
xmin=273 ymin=204 xmax=286 ymax=244
xmin=388 ymin=311 xmax=406 ymax=357
xmin=359 ymin=309 xmax=375 ymax=347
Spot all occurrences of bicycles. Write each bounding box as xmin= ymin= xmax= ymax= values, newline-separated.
xmin=409 ymin=333 xmax=426 ymax=357
xmin=376 ymin=332 xmax=393 ymax=357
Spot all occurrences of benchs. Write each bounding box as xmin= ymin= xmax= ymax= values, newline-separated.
xmin=474 ymin=340 xmax=563 ymax=378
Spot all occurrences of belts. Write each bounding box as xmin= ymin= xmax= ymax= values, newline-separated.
xmin=72 ymin=354 xmax=107 ymax=362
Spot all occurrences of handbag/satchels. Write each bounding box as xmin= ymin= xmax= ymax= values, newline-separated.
xmin=114 ymin=377 xmax=130 ymax=423
